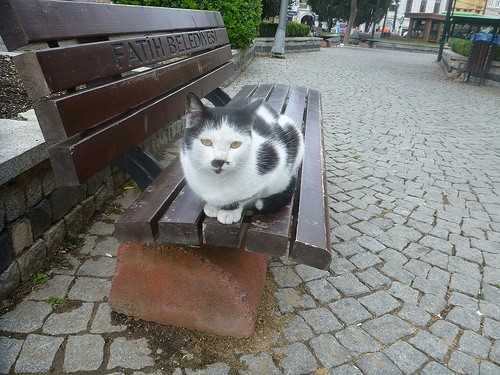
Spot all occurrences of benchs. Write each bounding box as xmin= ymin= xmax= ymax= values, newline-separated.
xmin=313 ymin=27 xmax=340 ymax=47
xmin=0 ymin=0 xmax=332 ymax=270
xmin=359 ymin=33 xmax=380 ymax=48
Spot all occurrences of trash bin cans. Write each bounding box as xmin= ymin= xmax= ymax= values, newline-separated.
xmin=469 ymin=40 xmax=495 ymax=73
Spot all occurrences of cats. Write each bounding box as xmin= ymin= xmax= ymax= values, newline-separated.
xmin=179 ymin=91 xmax=306 ymax=225
